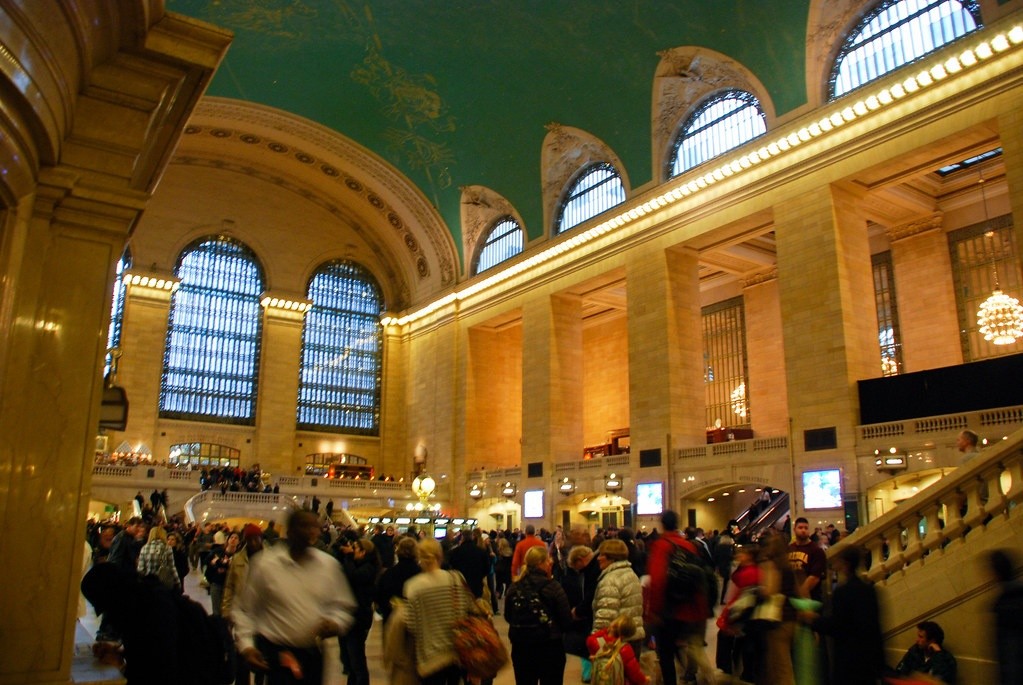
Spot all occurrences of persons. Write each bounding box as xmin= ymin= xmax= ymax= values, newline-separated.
xmin=979 ymin=549 xmax=1023 ymax=685
xmin=80 ymin=450 xmax=893 ymax=685
xmin=893 ymin=621 xmax=958 ymax=685
xmin=955 ymin=430 xmax=994 ymax=533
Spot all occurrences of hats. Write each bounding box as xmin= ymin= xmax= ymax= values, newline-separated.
xmin=81 ymin=560 xmax=126 ymax=617
xmin=243 ymin=523 xmax=262 ymax=540
xmin=213 ymin=531 xmax=226 ymax=545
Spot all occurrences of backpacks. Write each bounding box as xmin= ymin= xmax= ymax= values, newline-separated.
xmin=588 ymin=636 xmax=628 ymax=685
xmin=661 ymin=535 xmax=707 ymax=618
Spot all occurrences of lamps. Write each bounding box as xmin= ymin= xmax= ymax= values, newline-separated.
xmin=412 ymin=469 xmax=437 ymax=510
xmin=219 ymin=219 xmax=235 ymax=241
xmin=975 ymin=156 xmax=1023 ymax=345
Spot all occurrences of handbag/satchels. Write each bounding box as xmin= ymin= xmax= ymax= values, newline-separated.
xmin=448 ymin=569 xmax=507 ymax=685
xmin=750 ymin=593 xmax=786 ymax=622
xmin=580 ymin=657 xmax=592 ymax=683
xmin=727 ymin=586 xmax=758 ymax=624
xmin=155 ymin=543 xmax=178 ymax=590
